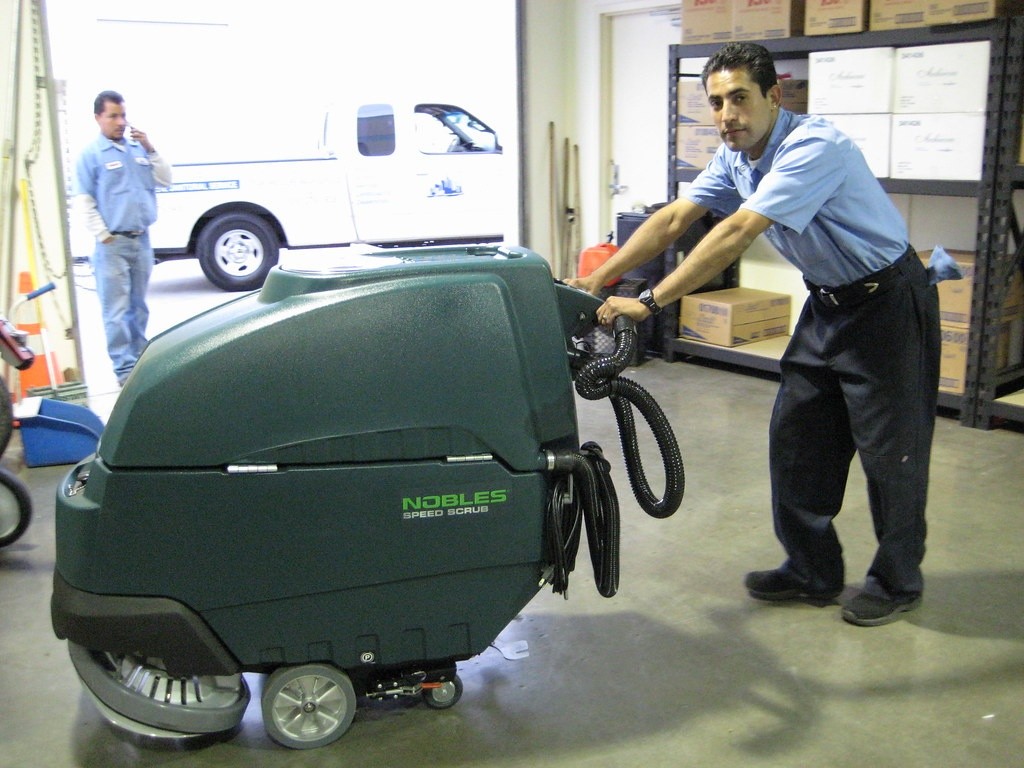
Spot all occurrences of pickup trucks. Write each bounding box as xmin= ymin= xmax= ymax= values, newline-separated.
xmin=72 ymin=88 xmax=512 ymax=293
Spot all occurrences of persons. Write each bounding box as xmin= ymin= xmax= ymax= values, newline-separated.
xmin=67 ymin=88 xmax=174 ymax=386
xmin=558 ymin=41 xmax=943 ymax=628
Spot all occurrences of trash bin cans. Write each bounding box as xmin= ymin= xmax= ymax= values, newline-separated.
xmin=616 ymin=212 xmax=736 ymax=352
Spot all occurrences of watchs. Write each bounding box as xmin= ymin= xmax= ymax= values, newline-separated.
xmin=637 ymin=288 xmax=665 ymax=316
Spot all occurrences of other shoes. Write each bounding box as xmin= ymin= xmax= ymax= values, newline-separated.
xmin=119 ymin=379 xmax=126 ymax=386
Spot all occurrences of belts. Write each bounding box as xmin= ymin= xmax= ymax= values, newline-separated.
xmin=815 ymin=246 xmax=916 ymax=307
xmin=110 ymin=230 xmax=147 ymax=239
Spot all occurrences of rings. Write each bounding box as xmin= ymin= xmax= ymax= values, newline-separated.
xmin=602 ymin=316 xmax=606 ymax=320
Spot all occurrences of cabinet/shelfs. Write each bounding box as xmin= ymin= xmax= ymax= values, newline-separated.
xmin=669 ymin=16 xmax=1009 ymax=425
xmin=972 ymin=14 xmax=1024 ymax=429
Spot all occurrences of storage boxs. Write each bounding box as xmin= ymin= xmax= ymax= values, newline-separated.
xmin=915 ymin=247 xmax=1024 ymax=396
xmin=676 ymin=285 xmax=791 ymax=347
xmin=675 ymin=0 xmax=1002 ymax=181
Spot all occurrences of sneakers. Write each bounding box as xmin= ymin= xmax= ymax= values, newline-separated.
xmin=841 ymin=591 xmax=922 ymax=625
xmin=746 ymin=569 xmax=842 ymax=599
